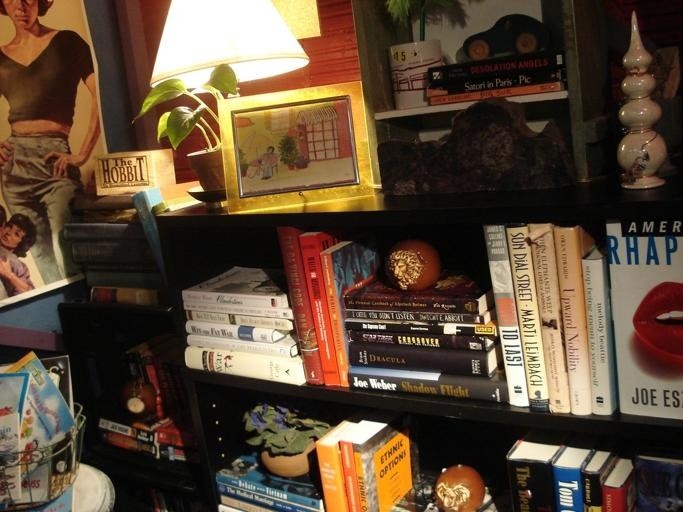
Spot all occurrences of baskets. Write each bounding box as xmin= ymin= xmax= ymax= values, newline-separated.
xmin=0 ymin=402 xmax=88 ymax=512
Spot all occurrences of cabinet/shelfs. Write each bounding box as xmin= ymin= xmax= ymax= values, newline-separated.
xmin=350 ymin=0 xmax=683 ymax=192
xmin=58 ymin=207 xmax=683 ymax=512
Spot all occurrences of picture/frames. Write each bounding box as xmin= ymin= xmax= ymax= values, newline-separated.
xmin=216 ymin=81 xmax=376 ymax=214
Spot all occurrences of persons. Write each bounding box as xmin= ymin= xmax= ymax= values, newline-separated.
xmin=0 ymin=0 xmax=101 ymax=284
xmin=258 ymin=146 xmax=280 ymax=180
xmin=0 ymin=205 xmax=26 ymax=299
xmin=0 ymin=213 xmax=37 ymax=295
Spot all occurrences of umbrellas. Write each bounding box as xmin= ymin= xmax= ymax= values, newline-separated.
xmin=240 ymin=132 xmax=273 ymax=175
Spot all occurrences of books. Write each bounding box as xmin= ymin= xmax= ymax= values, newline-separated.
xmin=424 ymin=52 xmax=567 ymax=105
xmin=0 ymin=216 xmax=681 ymax=511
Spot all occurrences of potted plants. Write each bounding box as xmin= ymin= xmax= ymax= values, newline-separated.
xmin=242 ymin=403 xmax=330 ymax=478
xmin=132 ymin=66 xmax=240 ymax=193
xmin=383 ymin=1 xmax=470 ymax=110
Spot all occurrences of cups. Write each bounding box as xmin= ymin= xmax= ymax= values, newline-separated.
xmin=386 ymin=39 xmax=446 ymax=111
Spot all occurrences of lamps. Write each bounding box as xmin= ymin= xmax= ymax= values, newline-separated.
xmin=149 ymin=0 xmax=310 ymax=95
